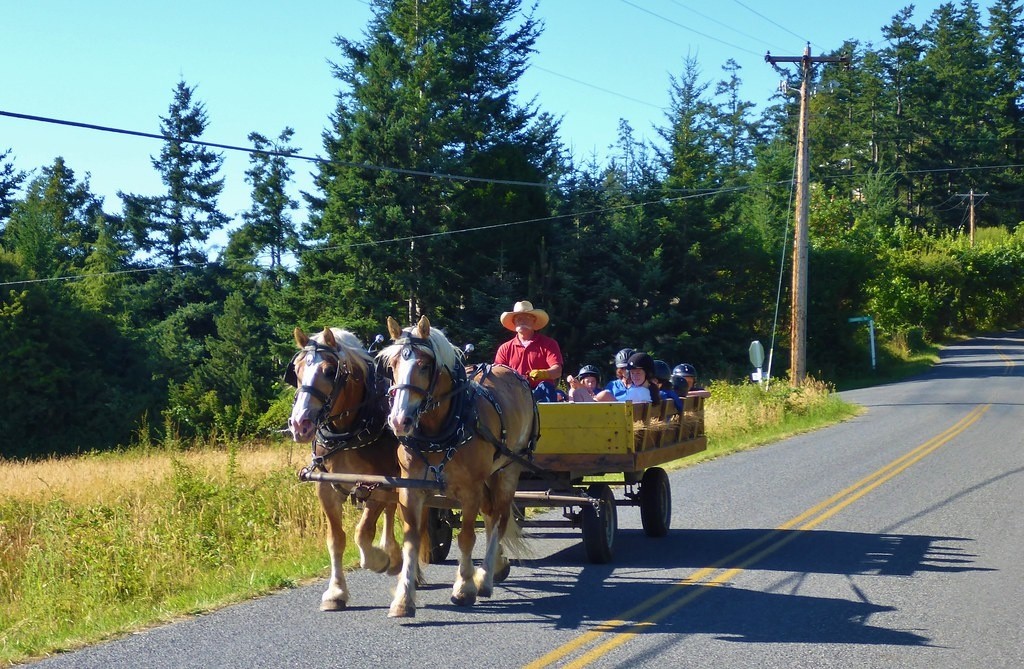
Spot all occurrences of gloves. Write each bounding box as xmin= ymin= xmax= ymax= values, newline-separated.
xmin=528 ymin=370 xmax=549 ymax=386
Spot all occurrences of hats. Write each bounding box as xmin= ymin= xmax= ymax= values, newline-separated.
xmin=500 ymin=301 xmax=549 ymax=331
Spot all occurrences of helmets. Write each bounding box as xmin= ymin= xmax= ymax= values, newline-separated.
xmin=653 ymin=360 xmax=670 ymax=380
xmin=615 ymin=348 xmax=637 ymax=368
xmin=626 ymin=353 xmax=655 ymax=387
xmin=579 ymin=365 xmax=601 ymax=383
xmin=669 ymin=375 xmax=688 ymax=396
xmin=673 ymin=363 xmax=697 ymax=382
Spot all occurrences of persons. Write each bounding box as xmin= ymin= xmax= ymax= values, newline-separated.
xmin=494 ymin=300 xmax=563 ymax=401
xmin=567 ymin=348 xmax=711 ymax=413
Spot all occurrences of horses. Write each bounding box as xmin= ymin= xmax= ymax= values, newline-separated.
xmin=287 ymin=315 xmax=540 ymax=619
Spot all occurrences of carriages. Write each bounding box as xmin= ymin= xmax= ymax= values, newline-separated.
xmin=287 ymin=314 xmax=712 ymax=619
